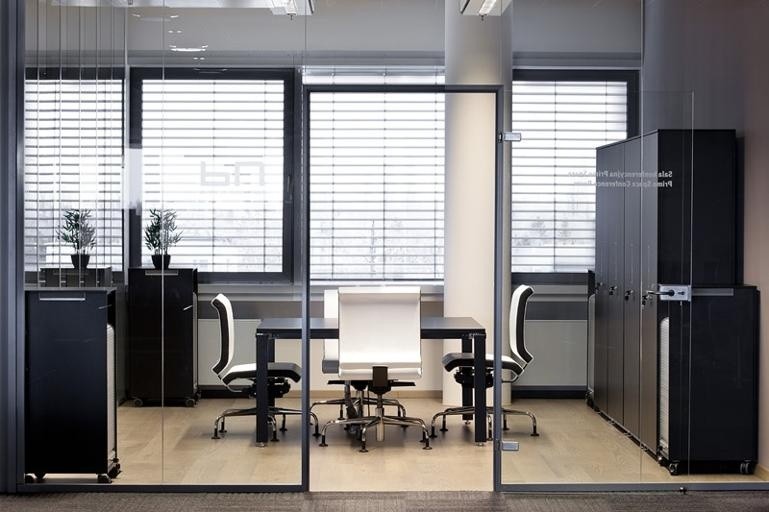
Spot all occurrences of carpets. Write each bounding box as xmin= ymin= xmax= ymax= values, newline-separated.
xmin=33 ymin=399 xmax=769 ymax=493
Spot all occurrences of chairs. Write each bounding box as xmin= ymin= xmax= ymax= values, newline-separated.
xmin=211 ymin=284 xmax=538 ymax=452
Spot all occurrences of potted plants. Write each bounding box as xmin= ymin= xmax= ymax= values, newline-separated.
xmin=58 ymin=209 xmax=96 ymax=269
xmin=143 ymin=207 xmax=182 ymax=269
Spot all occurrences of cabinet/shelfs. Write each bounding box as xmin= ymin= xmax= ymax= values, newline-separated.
xmin=40 ymin=266 xmax=113 ymax=288
xmin=24 ymin=287 xmax=122 ymax=484
xmin=128 ymin=268 xmax=201 ymax=408
xmin=585 ymin=129 xmax=757 ymax=473
